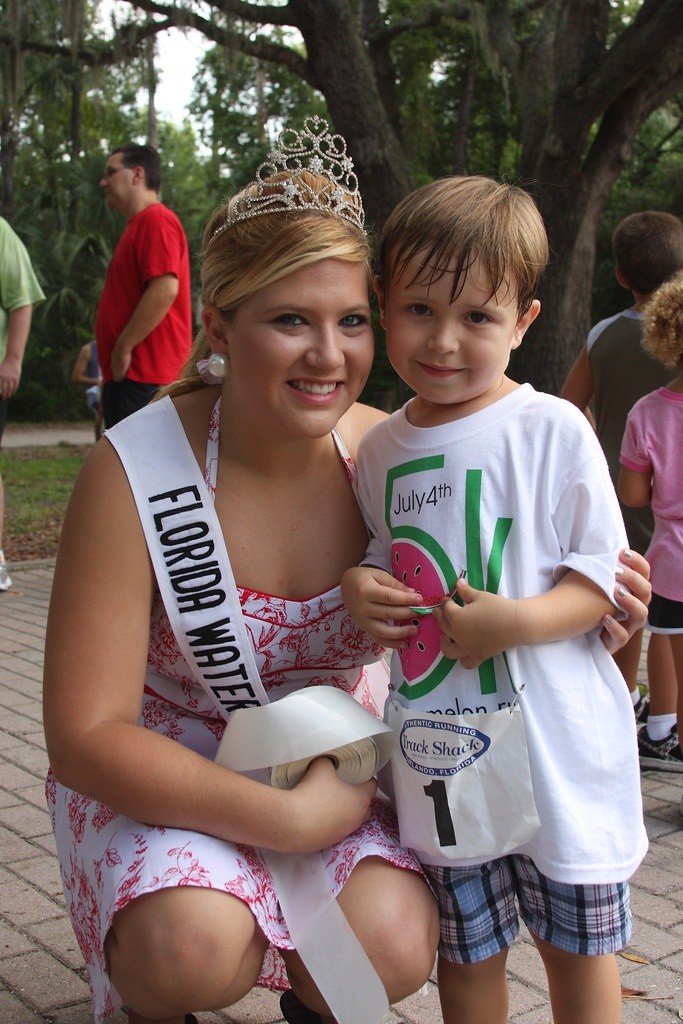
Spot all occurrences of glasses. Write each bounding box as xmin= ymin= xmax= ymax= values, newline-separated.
xmin=102 ymin=166 xmax=128 ymax=179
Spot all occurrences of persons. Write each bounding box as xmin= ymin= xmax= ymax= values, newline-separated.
xmin=0 ymin=217 xmax=47 ymax=592
xmin=95 ymin=144 xmax=192 ymax=428
xmin=616 ymin=272 xmax=683 ymax=746
xmin=41 ymin=171 xmax=653 ymax=1024
xmin=336 ymin=176 xmax=648 ymax=1024
xmin=71 ymin=339 xmax=106 ymax=438
xmin=560 ymin=211 xmax=683 ymax=774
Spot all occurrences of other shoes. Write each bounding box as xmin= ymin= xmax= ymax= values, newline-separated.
xmin=637 ymin=723 xmax=683 ymax=772
xmin=634 ymin=696 xmax=652 ymax=724
xmin=0 ymin=567 xmax=12 ymax=591
